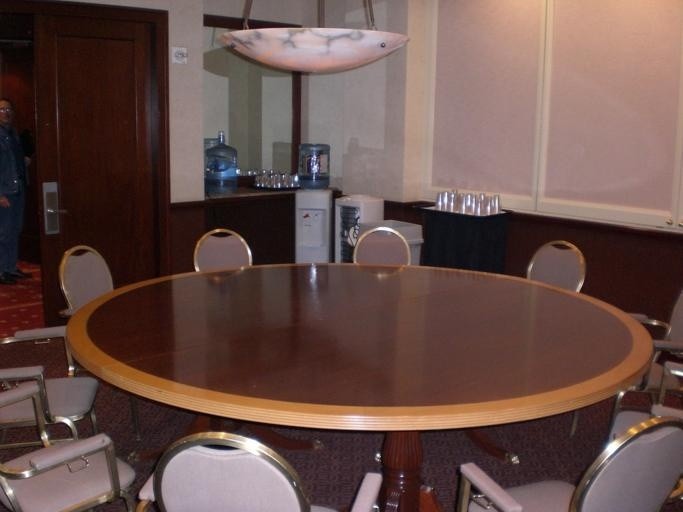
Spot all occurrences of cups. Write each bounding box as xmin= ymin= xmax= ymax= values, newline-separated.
xmin=253 ymin=168 xmax=299 ymax=189
xmin=239 ymin=168 xmax=261 ymax=177
xmin=435 ymin=190 xmax=501 ymax=216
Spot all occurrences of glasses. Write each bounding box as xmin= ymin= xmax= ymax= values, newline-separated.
xmin=0 ymin=108 xmax=14 ymax=113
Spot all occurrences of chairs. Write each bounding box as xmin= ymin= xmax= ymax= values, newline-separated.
xmin=190 ymin=227 xmax=253 ymax=272
xmin=0 ymin=377 xmax=136 ymax=510
xmin=569 ymin=287 xmax=683 ymax=431
xmin=57 ymin=244 xmax=143 ymax=441
xmin=349 ymin=225 xmax=415 ymax=266
xmin=452 ymin=415 xmax=682 ymax=512
xmin=607 ymin=364 xmax=683 ymax=500
xmin=0 ymin=324 xmax=100 ymax=440
xmin=134 ymin=429 xmax=384 ymax=512
xmin=526 ymin=238 xmax=588 ymax=294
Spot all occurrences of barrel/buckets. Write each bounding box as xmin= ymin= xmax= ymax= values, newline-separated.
xmin=203 ymin=129 xmax=238 ymax=195
xmin=295 ymin=141 xmax=332 ymax=191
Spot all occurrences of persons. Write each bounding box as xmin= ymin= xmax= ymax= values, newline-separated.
xmin=0 ymin=98 xmax=37 ymax=285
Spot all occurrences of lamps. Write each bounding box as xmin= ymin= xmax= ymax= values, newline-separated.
xmin=217 ymin=1 xmax=411 ymax=78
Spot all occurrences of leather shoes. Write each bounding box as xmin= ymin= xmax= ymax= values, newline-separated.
xmin=0 ymin=272 xmax=17 ymax=284
xmin=4 ymin=269 xmax=32 ymax=278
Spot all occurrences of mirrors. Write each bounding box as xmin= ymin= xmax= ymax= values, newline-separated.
xmin=201 ymin=13 xmax=302 ymax=186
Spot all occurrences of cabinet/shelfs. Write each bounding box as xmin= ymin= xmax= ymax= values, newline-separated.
xmin=207 ymin=186 xmax=297 ymax=264
xmin=417 ymin=207 xmax=512 ymax=273
